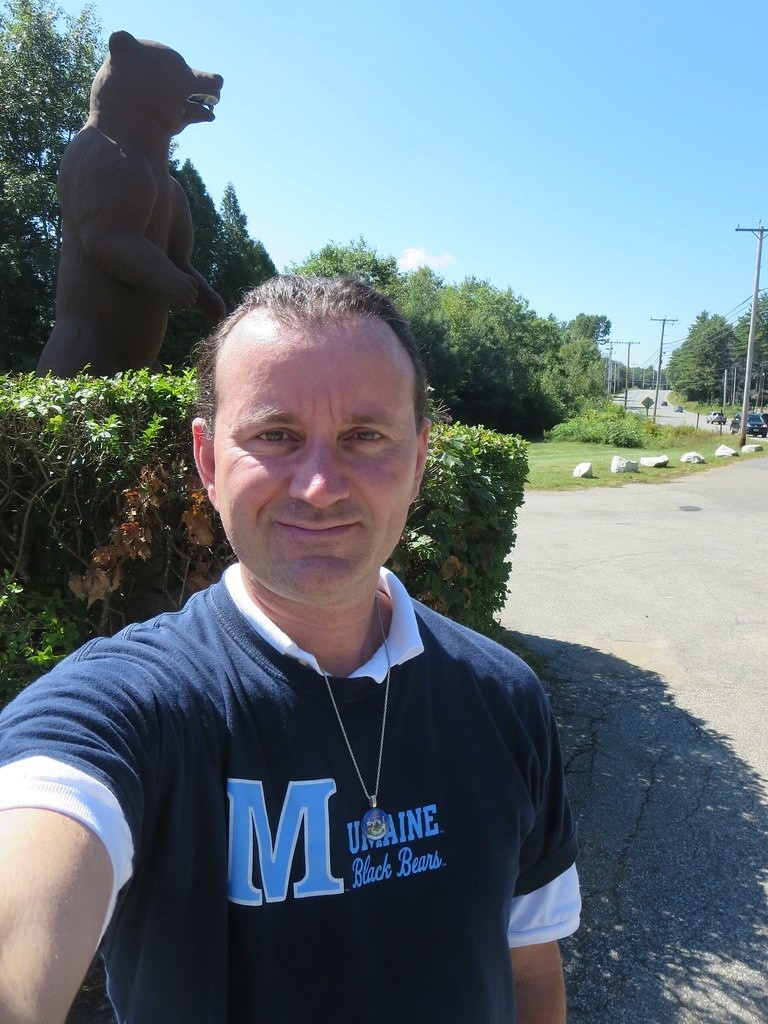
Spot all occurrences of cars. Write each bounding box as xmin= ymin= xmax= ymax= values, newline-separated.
xmin=730 ymin=413 xmax=768 ymax=438
xmin=674 ymin=406 xmax=683 ymax=412
xmin=662 ymin=402 xmax=667 ymax=406
xmin=706 ymin=411 xmax=727 ymax=424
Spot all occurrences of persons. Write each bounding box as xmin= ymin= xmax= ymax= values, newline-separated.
xmin=0 ymin=277 xmax=581 ymax=1024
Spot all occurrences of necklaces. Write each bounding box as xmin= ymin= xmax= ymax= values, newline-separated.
xmin=319 ymin=593 xmax=391 ymax=839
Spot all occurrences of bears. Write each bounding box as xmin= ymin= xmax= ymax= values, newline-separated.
xmin=33 ymin=30 xmax=230 ymax=394
